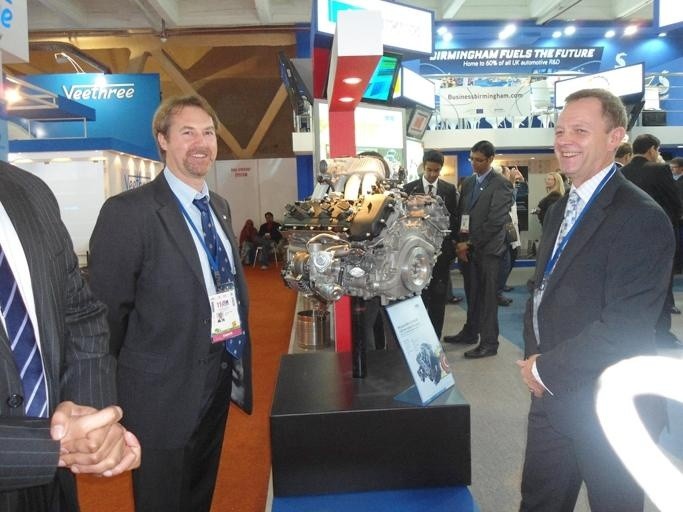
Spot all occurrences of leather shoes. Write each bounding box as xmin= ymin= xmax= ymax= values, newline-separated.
xmin=448 ymin=295 xmax=462 ymax=303
xmin=464 ymin=345 xmax=497 ymax=357
xmin=496 ymin=285 xmax=514 ymax=306
xmin=657 ymin=339 xmax=683 ymax=348
xmin=443 ymin=331 xmax=478 ymax=344
xmin=671 ymin=305 xmax=681 ymax=314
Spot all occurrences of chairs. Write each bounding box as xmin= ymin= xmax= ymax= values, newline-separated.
xmin=251 ymin=237 xmax=287 ymax=268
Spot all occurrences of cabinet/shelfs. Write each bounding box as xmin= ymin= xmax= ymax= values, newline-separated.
xmin=262 ymin=289 xmax=485 ymax=512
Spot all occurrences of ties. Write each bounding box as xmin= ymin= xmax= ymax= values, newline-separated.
xmin=556 ymin=191 xmax=580 ymax=262
xmin=0 ymin=244 xmax=47 ymax=418
xmin=191 ymin=195 xmax=247 ymax=360
xmin=428 ymin=185 xmax=432 ymax=192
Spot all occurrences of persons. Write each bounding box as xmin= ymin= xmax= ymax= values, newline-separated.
xmin=402 ymin=154 xmax=459 ymax=343
xmin=439 ymin=168 xmax=572 ymax=304
xmin=241 ymin=220 xmax=263 ymax=266
xmin=443 ymin=140 xmax=513 ymax=360
xmin=1 ymin=150 xmax=141 ymax=511
xmin=84 ymin=94 xmax=257 ymax=511
xmin=515 ymin=87 xmax=677 ymax=511
xmin=616 ymin=140 xmax=683 ymax=312
xmin=619 ymin=134 xmax=681 ymax=349
xmin=259 ymin=211 xmax=282 ymax=266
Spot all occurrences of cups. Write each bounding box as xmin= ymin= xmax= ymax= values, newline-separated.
xmin=296 ymin=310 xmax=331 ymax=349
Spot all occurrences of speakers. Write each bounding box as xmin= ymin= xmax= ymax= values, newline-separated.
xmin=638 ymin=110 xmax=668 ymax=127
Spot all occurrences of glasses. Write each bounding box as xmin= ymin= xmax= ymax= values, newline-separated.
xmin=467 ymin=158 xmax=487 ymax=163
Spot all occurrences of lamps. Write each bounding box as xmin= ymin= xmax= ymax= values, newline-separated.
xmin=159 ymin=19 xmax=168 ymax=43
